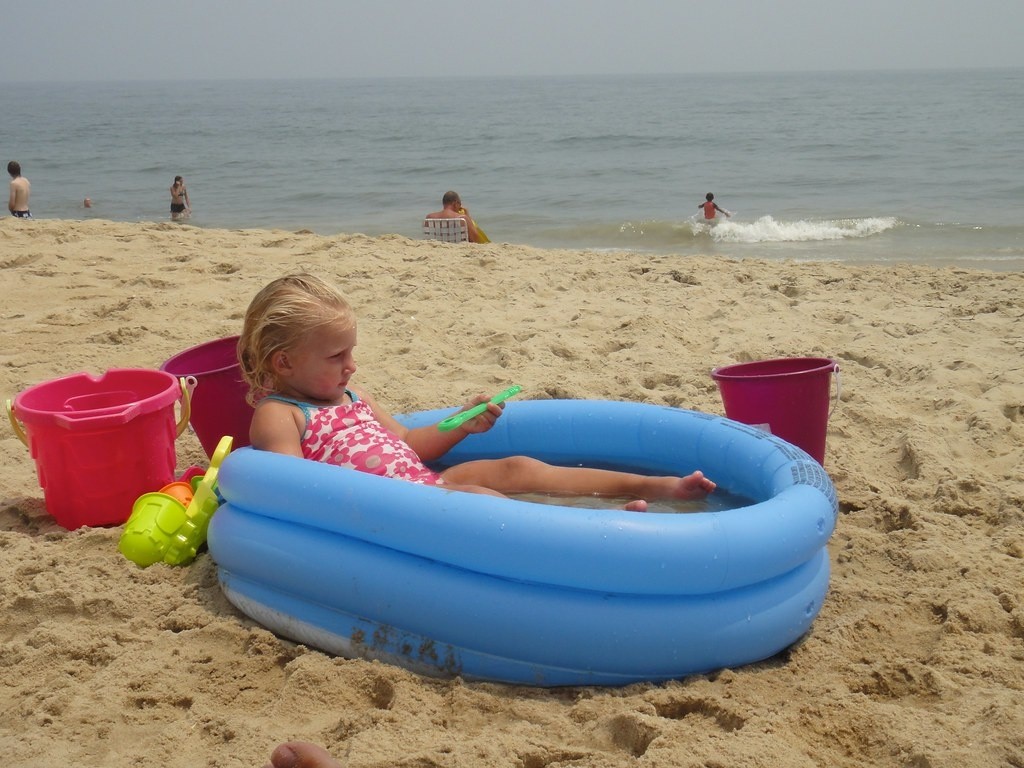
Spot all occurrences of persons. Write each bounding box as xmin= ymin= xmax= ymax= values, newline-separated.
xmin=698 ymin=192 xmax=731 ymax=219
xmin=171 ymin=176 xmax=191 ymax=218
xmin=7 ymin=161 xmax=32 ymax=218
xmin=425 ymin=191 xmax=478 ymax=242
xmin=235 ymin=274 xmax=716 ymax=511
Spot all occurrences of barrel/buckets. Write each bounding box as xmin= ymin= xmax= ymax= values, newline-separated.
xmin=712 ymin=357 xmax=842 ymax=467
xmin=6 ymin=368 xmax=191 ymax=531
xmin=159 ymin=481 xmax=195 ymax=508
xmin=116 ymin=492 xmax=187 ymax=568
xmin=159 ymin=335 xmax=272 ymax=462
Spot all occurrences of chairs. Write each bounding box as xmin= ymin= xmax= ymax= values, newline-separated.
xmin=423 ymin=218 xmax=468 ymax=243
